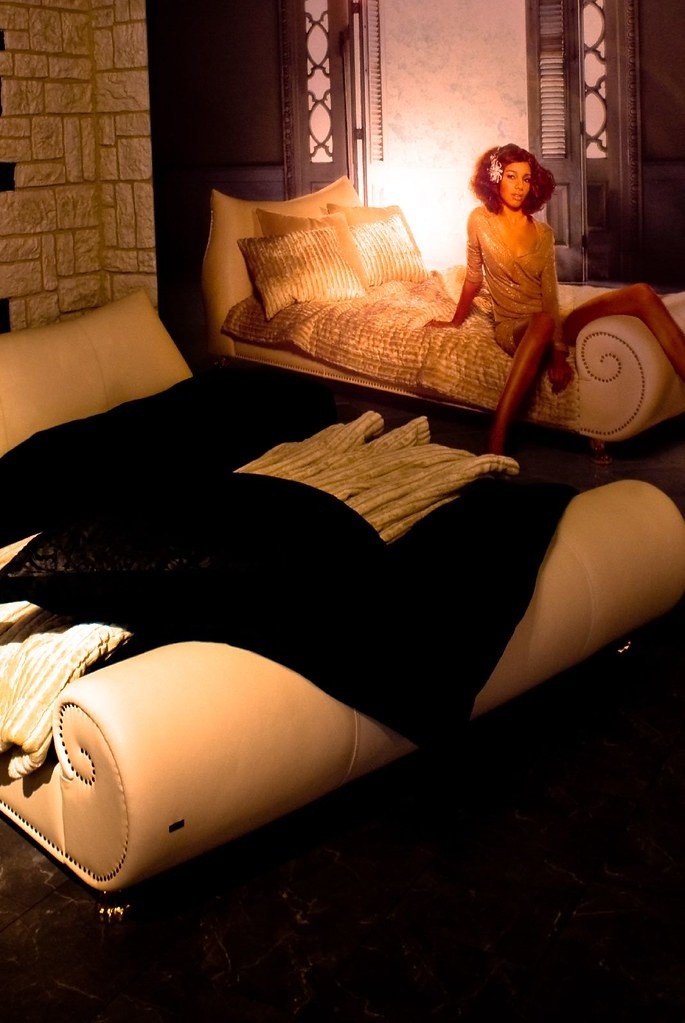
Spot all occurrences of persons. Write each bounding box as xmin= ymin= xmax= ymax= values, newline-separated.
xmin=421 ymin=143 xmax=685 ymax=455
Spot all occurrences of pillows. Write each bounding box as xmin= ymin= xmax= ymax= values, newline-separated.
xmin=237 ymin=226 xmax=366 ymax=322
xmin=254 ymin=206 xmax=371 ymax=288
xmin=324 ymin=201 xmax=427 ymax=286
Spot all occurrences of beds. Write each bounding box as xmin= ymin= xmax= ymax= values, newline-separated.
xmin=200 ymin=174 xmax=685 ymax=467
xmin=0 ymin=289 xmax=685 ymax=893
